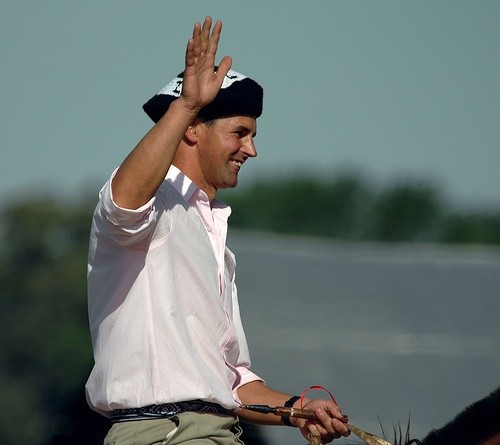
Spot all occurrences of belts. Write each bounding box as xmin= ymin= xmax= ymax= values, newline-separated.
xmin=110 ymin=399 xmax=234 ymax=424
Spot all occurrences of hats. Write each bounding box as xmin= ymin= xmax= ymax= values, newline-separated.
xmin=142 ymin=66 xmax=263 ymax=123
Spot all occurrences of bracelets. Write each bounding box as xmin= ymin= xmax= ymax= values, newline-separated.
xmin=282 ymin=395 xmax=301 ymax=427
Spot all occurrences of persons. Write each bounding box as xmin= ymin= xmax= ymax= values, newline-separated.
xmin=85 ymin=16 xmax=351 ymax=445
